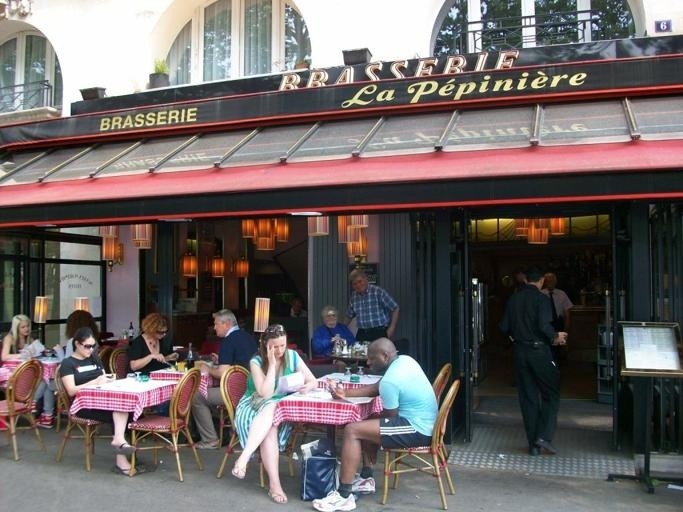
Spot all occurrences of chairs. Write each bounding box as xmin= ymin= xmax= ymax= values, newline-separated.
xmin=128 ymin=369 xmax=203 ymax=481
xmin=0 ymin=360 xmax=47 ymax=461
xmin=381 ymin=380 xmax=460 ymax=510
xmin=433 ymin=363 xmax=452 ymax=409
xmin=110 ymin=347 xmax=130 ymax=380
xmin=56 ymin=362 xmax=106 ymax=471
xmin=216 ymin=365 xmax=298 ymax=489
xmin=54 ymin=364 xmax=71 ymax=432
xmin=214 ymin=402 xmax=234 ymax=447
xmin=97 ymin=346 xmax=116 ymax=375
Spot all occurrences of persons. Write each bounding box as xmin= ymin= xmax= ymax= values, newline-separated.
xmin=126 ymin=313 xmax=198 ymax=443
xmin=20 ymin=310 xmax=99 ymax=429
xmin=313 ymin=338 xmax=439 ymax=512
xmin=287 ymin=295 xmax=305 ymax=323
xmin=538 ymin=271 xmax=570 ymax=374
xmin=312 ymin=306 xmax=356 ymax=374
xmin=58 ymin=327 xmax=137 ymax=476
xmin=503 ymin=267 xmax=566 ymax=456
xmin=0 ymin=316 xmax=36 ymax=362
xmin=189 ymin=309 xmax=261 ymax=449
xmin=229 ymin=325 xmax=315 ymax=502
xmin=340 ymin=268 xmax=400 ymax=344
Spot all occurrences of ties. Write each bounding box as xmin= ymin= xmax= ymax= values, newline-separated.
xmin=550 ymin=290 xmax=558 ymax=325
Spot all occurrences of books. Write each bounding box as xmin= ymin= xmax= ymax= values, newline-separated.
xmin=329 ymin=384 xmax=374 ymax=405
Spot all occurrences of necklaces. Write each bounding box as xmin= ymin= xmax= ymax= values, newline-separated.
xmin=147 ymin=339 xmax=158 ymax=350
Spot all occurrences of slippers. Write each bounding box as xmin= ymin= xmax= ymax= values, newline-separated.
xmin=195 ymin=441 xmax=221 ymax=451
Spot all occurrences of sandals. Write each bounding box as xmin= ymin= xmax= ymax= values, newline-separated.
xmin=112 ymin=438 xmax=136 ymax=455
xmin=116 ymin=462 xmax=136 ymax=477
xmin=232 ymin=459 xmax=246 ymax=479
xmin=268 ymin=489 xmax=288 ymax=504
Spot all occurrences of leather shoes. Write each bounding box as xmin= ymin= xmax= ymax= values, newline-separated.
xmin=535 ymin=437 xmax=557 ymax=455
xmin=531 ymin=445 xmax=543 ymax=454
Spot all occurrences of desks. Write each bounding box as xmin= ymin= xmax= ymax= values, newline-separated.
xmin=149 ymin=359 xmax=219 ymax=399
xmin=332 ymin=347 xmax=378 ymax=363
xmin=272 ymin=373 xmax=385 ymax=462
xmin=68 ymin=378 xmax=180 ymax=475
xmin=1 ymin=357 xmax=73 ymax=381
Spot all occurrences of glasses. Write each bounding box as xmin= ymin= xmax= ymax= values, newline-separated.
xmin=261 ymin=324 xmax=285 ymax=343
xmin=155 ymin=330 xmax=168 ymax=336
xmin=78 ymin=342 xmax=96 ymax=351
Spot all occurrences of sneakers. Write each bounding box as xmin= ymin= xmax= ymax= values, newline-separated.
xmin=312 ymin=491 xmax=356 ymax=508
xmin=23 ymin=399 xmax=38 ymax=413
xmin=32 ymin=413 xmax=54 ymax=429
xmin=352 ymin=473 xmax=376 ymax=496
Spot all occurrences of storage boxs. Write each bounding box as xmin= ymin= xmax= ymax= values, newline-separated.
xmin=258 ymin=219 xmax=276 ymax=238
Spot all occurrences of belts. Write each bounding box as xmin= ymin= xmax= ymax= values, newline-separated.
xmin=528 ymin=340 xmax=548 ymax=346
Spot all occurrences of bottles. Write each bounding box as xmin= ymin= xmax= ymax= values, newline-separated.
xmin=128 ymin=321 xmax=134 ymax=341
xmin=335 ymin=334 xmax=341 ymax=355
xmin=187 ymin=343 xmax=194 ymax=370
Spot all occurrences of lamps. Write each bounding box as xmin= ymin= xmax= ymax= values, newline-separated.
xmin=257 ymin=235 xmax=276 ymax=249
xmin=527 ymin=216 xmax=549 ymax=245
xmin=550 ymin=218 xmax=566 ymax=235
xmin=101 ymin=235 xmax=122 ymax=272
xmin=242 ymin=218 xmax=255 ymax=238
xmin=211 ymin=256 xmax=226 ymax=277
xmin=99 ymin=226 xmax=120 ymax=240
xmin=33 ymin=296 xmax=49 ymax=343
xmin=252 ymin=297 xmax=270 ymax=336
xmin=338 ymin=214 xmax=360 ymax=243
xmin=180 ymin=254 xmax=199 ymax=279
xmin=272 ymin=219 xmax=289 ymax=241
xmin=347 ymin=239 xmax=367 ymax=259
xmin=307 ymin=216 xmax=329 ymax=236
xmin=514 ymin=218 xmax=531 ymax=237
xmin=350 ymin=214 xmax=370 ymax=227
xmin=130 ymin=223 xmax=152 ymax=248
xmin=72 ymin=297 xmax=88 ymax=310
xmin=233 ymin=258 xmax=250 ymax=277
